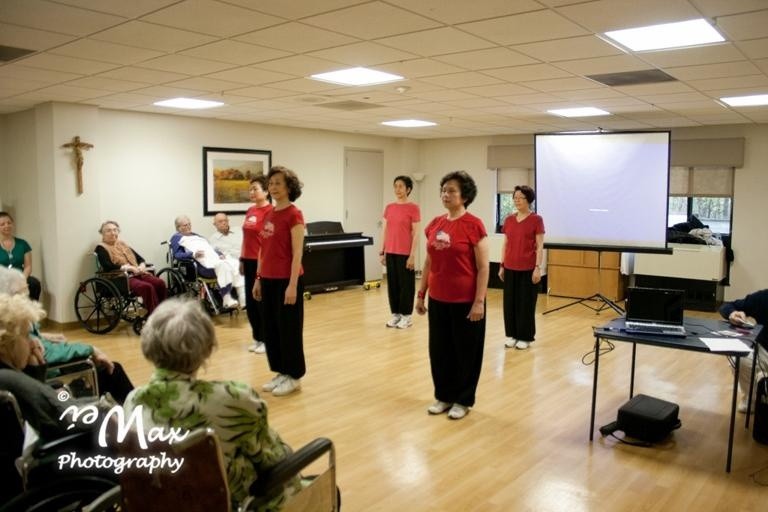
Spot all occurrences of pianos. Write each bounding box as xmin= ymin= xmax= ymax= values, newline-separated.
xmin=301 ymin=221 xmax=373 ymax=293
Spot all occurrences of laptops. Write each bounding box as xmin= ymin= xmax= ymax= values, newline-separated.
xmin=625 ymin=286 xmax=687 ymax=338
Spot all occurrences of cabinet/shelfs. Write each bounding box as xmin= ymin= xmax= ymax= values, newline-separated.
xmin=631 ymin=241 xmax=730 ymax=315
xmin=545 ymin=247 xmax=624 ymax=303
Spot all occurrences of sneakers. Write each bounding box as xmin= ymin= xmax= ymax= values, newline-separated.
xmin=386 ymin=314 xmax=400 ymax=327
xmin=272 ymin=377 xmax=301 ymax=397
xmin=222 ymin=294 xmax=247 ymax=310
xmin=504 ymin=336 xmax=517 ymax=348
xmin=248 ymin=341 xmax=266 ymax=353
xmin=739 ymin=400 xmax=754 ymax=413
xmin=394 ymin=315 xmax=413 ymax=329
xmin=514 ymin=340 xmax=529 ymax=349
xmin=447 ymin=403 xmax=469 ymax=419
xmin=261 ymin=374 xmax=288 ymax=391
xmin=427 ymin=401 xmax=450 ymax=414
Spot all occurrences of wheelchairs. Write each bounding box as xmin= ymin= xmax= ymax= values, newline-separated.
xmin=74 ymin=247 xmax=171 ymax=338
xmin=155 ymin=239 xmax=242 ymax=321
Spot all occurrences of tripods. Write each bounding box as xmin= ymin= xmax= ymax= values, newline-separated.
xmin=541 ymin=253 xmax=624 ymax=319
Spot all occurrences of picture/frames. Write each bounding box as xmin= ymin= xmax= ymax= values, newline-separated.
xmin=201 ymin=145 xmax=274 ymax=216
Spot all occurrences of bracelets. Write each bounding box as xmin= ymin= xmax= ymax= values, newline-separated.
xmin=500 ymin=262 xmax=504 ymax=268
xmin=534 ymin=265 xmax=542 ymax=269
xmin=379 ymin=250 xmax=384 ymax=255
xmin=418 ymin=290 xmax=425 ymax=299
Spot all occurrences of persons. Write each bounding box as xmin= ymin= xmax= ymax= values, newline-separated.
xmin=497 ymin=184 xmax=546 ymax=350
xmin=377 ymin=176 xmax=421 ymax=329
xmin=238 ymin=175 xmax=275 ymax=354
xmin=719 ymin=288 xmax=768 ymax=414
xmin=252 ymin=166 xmax=304 ymax=396
xmin=415 ymin=170 xmax=490 ymax=419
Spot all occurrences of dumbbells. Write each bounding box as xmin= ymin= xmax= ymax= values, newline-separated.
xmin=364 ymin=282 xmax=380 ymax=290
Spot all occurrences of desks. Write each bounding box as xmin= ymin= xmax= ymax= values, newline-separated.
xmin=587 ymin=314 xmax=766 ymax=475
